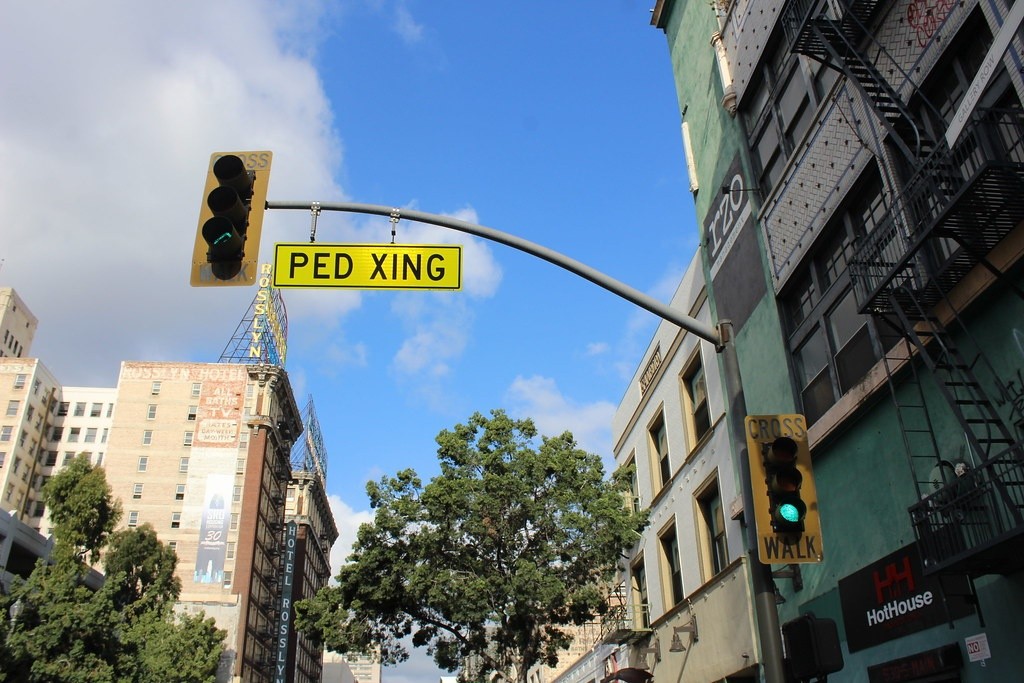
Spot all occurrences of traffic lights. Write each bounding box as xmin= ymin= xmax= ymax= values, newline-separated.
xmin=190 ymin=151 xmax=274 ymax=286
xmin=745 ymin=414 xmax=825 ymax=564
xmin=782 ymin=614 xmax=844 ymax=679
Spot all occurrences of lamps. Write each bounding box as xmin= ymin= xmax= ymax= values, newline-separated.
xmin=722 ymin=185 xmax=760 ymax=194
